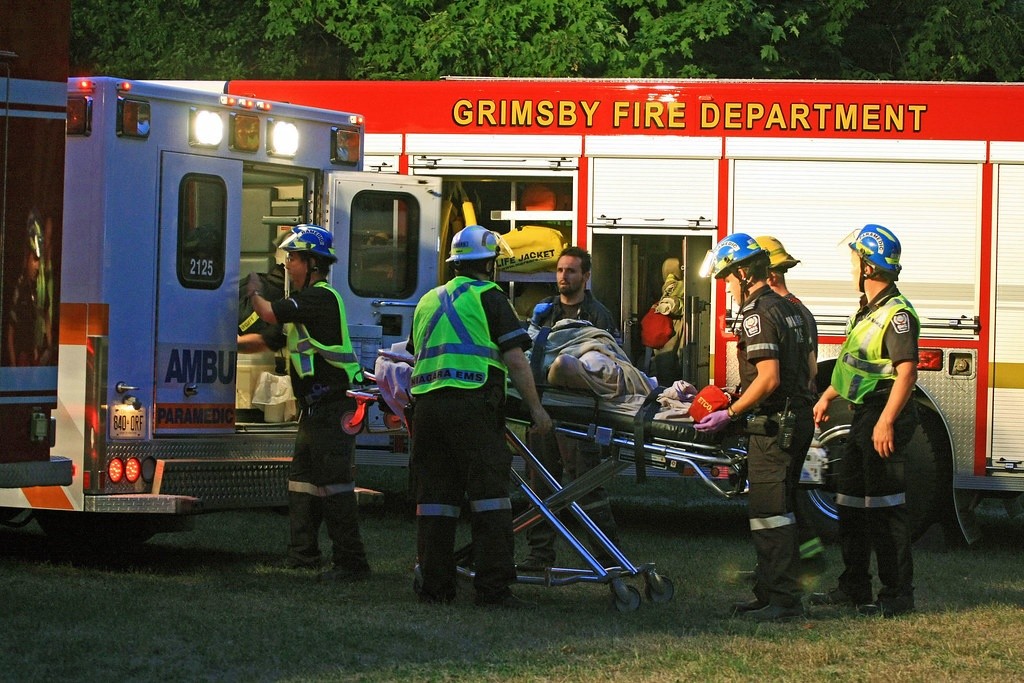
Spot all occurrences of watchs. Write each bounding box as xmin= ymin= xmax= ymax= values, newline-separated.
xmin=727 ymin=407 xmax=738 ymax=422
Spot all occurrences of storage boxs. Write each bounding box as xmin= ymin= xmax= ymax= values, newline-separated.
xmin=237 ymin=351 xmax=292 ymax=422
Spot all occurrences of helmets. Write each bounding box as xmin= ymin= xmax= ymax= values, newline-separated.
xmin=848 ymin=223 xmax=903 ymax=278
xmin=749 ymin=237 xmax=803 ymax=271
xmin=273 ymin=224 xmax=338 ymax=267
xmin=700 ymin=233 xmax=769 ymax=280
xmin=444 ymin=224 xmax=500 ymax=262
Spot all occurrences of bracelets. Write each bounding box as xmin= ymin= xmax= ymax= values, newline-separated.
xmin=248 ymin=289 xmax=265 ymax=298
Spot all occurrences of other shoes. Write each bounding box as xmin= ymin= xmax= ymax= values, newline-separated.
xmin=473 ymin=585 xmax=528 ymax=610
xmin=263 ymin=554 xmax=321 ymax=569
xmin=316 ymin=565 xmax=372 ymax=586
xmin=413 ymin=578 xmax=457 ymax=606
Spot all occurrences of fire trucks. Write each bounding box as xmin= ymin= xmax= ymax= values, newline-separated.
xmin=83 ymin=73 xmax=1023 ymax=549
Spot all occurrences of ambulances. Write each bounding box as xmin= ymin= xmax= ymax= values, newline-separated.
xmin=0 ymin=50 xmax=445 ymax=561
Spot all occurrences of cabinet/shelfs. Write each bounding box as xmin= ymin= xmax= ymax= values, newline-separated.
xmin=490 ymin=182 xmax=577 ymax=306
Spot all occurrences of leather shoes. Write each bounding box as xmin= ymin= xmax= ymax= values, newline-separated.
xmin=738 ymin=603 xmax=804 ymax=622
xmin=732 ymin=598 xmax=769 ymax=613
xmin=860 ymin=599 xmax=915 ymax=618
xmin=807 ymin=586 xmax=856 ymax=607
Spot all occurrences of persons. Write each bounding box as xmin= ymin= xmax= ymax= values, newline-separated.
xmin=812 ymin=223 xmax=923 ymax=617
xmin=405 ymin=225 xmax=554 ymax=612
xmin=693 ymin=233 xmax=819 ymax=625
xmin=547 ymin=354 xmax=588 ymax=390
xmin=526 ymin=246 xmax=625 ymax=353
xmin=9 ymin=212 xmax=51 ymax=461
xmin=245 ymin=225 xmax=375 ymax=585
